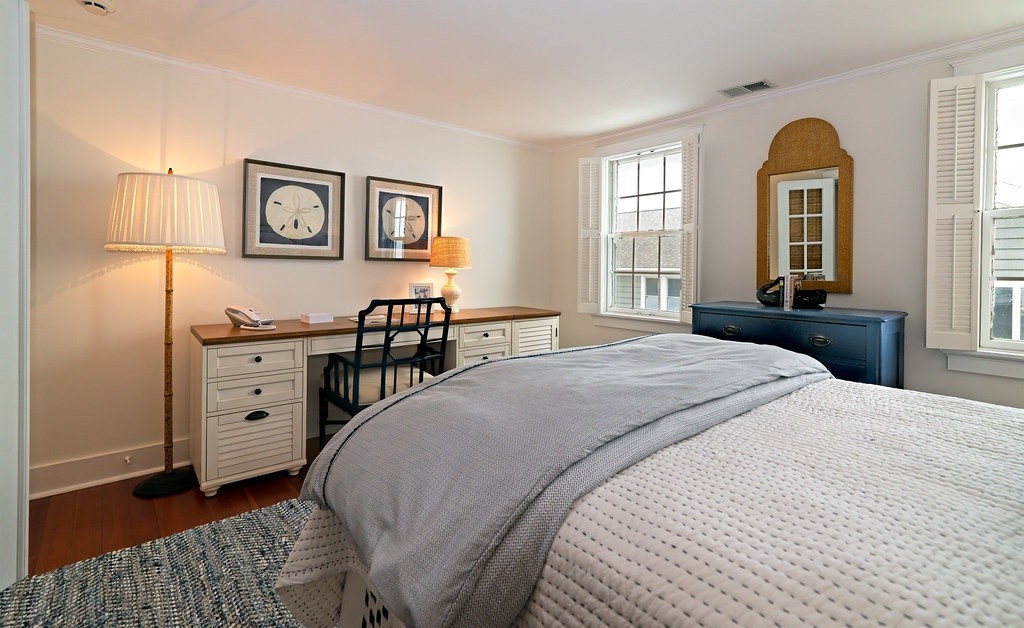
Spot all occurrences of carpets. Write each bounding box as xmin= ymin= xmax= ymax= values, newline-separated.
xmin=0 ymin=495 xmax=316 ymax=628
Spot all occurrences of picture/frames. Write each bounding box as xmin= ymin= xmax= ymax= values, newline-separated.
xmin=409 ymin=283 xmax=434 ymax=315
xmin=364 ymin=174 xmax=443 ymax=262
xmin=240 ymin=156 xmax=346 ymax=262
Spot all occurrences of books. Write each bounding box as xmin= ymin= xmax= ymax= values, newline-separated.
xmin=784 ymin=275 xmax=798 ymax=312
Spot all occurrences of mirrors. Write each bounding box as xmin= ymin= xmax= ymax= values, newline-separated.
xmin=755 ymin=118 xmax=854 ymax=294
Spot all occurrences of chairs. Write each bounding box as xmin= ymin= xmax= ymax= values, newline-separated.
xmin=315 ymin=296 xmax=454 ymax=450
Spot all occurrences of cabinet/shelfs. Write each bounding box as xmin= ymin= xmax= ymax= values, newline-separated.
xmin=184 ymin=303 xmax=565 ymax=498
xmin=687 ymin=299 xmax=909 ymax=390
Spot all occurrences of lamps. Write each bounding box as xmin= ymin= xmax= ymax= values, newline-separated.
xmin=429 ymin=235 xmax=472 ymax=315
xmin=104 ymin=163 xmax=228 ymax=503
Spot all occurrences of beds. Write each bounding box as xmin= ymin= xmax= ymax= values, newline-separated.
xmin=269 ymin=323 xmax=1024 ymax=628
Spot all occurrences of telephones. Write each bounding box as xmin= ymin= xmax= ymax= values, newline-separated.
xmin=225 ymin=306 xmax=275 ymax=327
